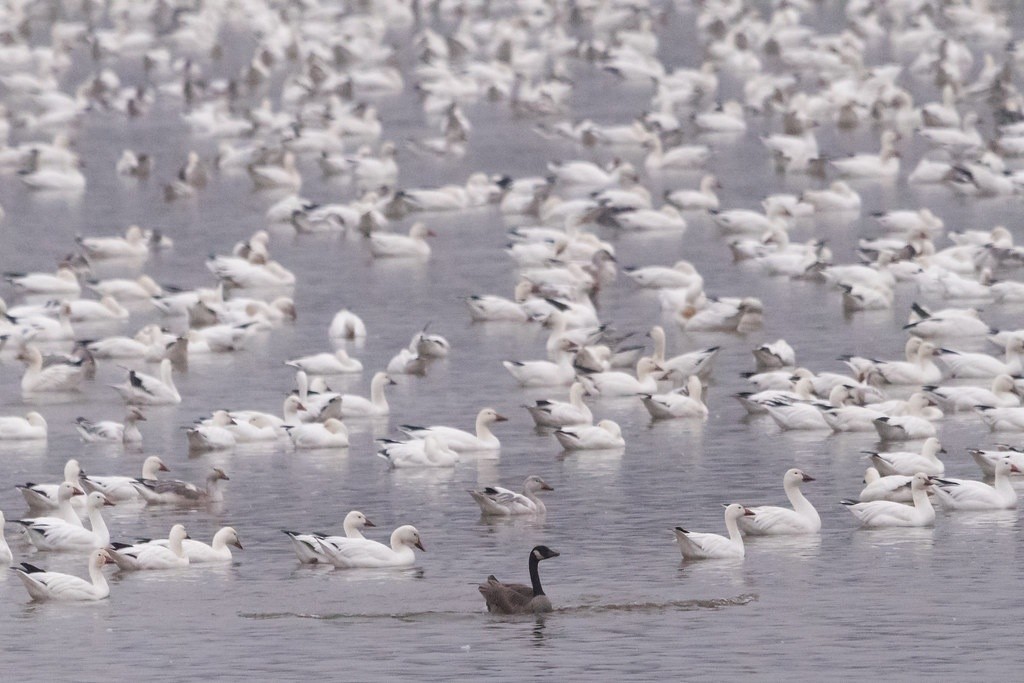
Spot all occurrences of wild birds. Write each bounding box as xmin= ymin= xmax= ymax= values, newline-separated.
xmin=1 ymin=1 xmax=1024 ymax=620
